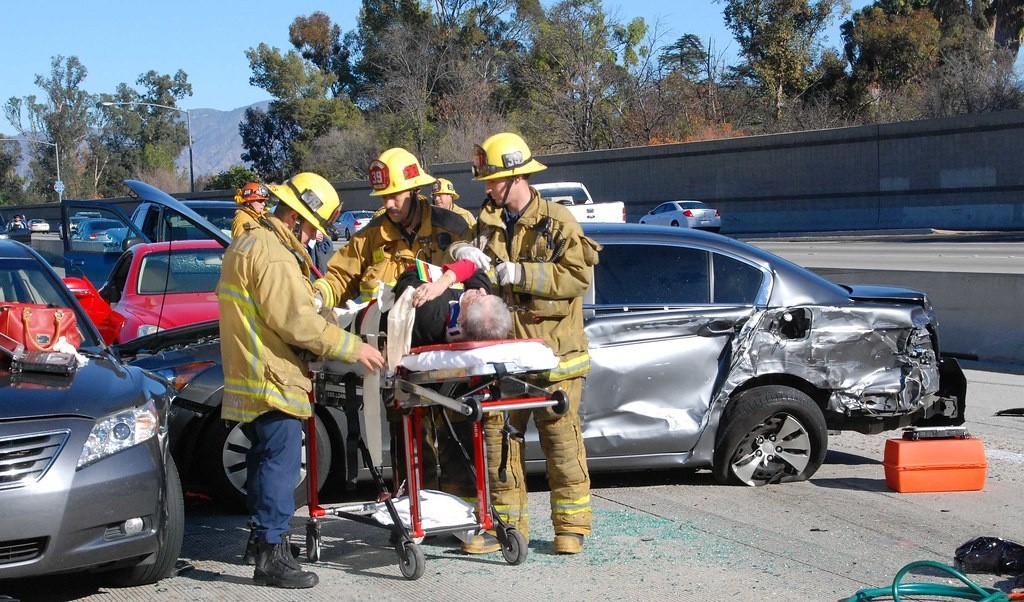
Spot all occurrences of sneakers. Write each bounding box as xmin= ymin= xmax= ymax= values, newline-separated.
xmin=554 ymin=533 xmax=583 ymax=554
xmin=463 ymin=531 xmax=528 ymax=554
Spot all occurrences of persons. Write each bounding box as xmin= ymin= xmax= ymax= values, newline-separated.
xmin=10 ymin=214 xmax=27 ymax=228
xmin=440 ymin=132 xmax=603 ymax=554
xmin=214 ymin=148 xmax=512 ymax=588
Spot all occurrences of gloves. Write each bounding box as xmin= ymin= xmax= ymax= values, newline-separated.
xmin=496 ymin=262 xmax=522 ymax=286
xmin=314 ymin=298 xmax=322 ymax=312
xmin=454 ymin=246 xmax=492 ymax=273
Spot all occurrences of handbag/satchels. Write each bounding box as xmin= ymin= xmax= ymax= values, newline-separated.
xmin=0 ymin=302 xmax=85 ymax=361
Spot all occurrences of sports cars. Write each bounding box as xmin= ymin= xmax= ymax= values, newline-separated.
xmin=30 ymin=240 xmax=224 ymax=346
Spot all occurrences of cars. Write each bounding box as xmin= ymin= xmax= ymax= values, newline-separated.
xmin=332 ymin=211 xmax=375 ymax=241
xmin=0 ymin=238 xmax=186 ymax=586
xmin=639 ymin=200 xmax=722 ymax=233
xmin=72 ymin=219 xmax=125 ymax=242
xmin=58 ymin=216 xmax=88 ymax=239
xmin=0 ymin=215 xmax=50 ymax=242
xmin=113 ymin=180 xmax=966 ymax=513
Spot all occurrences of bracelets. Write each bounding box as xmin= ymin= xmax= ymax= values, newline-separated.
xmin=442 ymin=273 xmax=454 ymax=287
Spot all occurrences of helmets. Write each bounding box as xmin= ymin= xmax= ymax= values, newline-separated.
xmin=430 ymin=178 xmax=459 ymax=200
xmin=472 ymin=133 xmax=547 ymax=180
xmin=264 ymin=172 xmax=344 ymax=238
xmin=368 ymin=148 xmax=436 ymax=196
xmin=241 ymin=183 xmax=270 ymax=201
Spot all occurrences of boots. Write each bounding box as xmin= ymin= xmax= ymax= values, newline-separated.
xmin=244 ymin=529 xmax=300 ymax=564
xmin=253 ymin=536 xmax=319 ymax=588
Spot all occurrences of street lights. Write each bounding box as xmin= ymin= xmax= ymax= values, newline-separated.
xmin=102 ymin=101 xmax=194 ymax=192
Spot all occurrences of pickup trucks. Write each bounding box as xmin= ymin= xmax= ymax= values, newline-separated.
xmin=528 ymin=182 xmax=626 ymax=224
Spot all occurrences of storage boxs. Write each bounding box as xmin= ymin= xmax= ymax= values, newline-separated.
xmin=883 ymin=438 xmax=987 ymax=492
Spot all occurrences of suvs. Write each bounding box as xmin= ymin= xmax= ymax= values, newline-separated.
xmin=61 ymin=199 xmax=241 ymax=291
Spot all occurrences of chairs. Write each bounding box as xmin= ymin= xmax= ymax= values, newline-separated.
xmin=140 ymin=260 xmax=179 ymax=294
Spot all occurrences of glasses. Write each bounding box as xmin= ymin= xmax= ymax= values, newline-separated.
xmin=256 ymin=199 xmax=264 ymax=203
